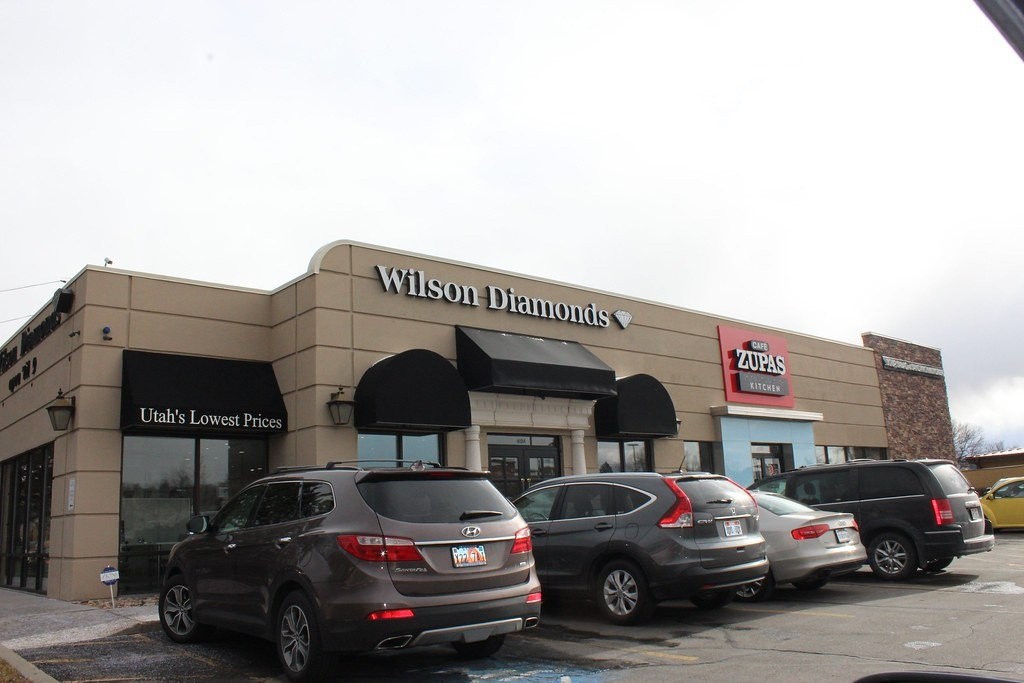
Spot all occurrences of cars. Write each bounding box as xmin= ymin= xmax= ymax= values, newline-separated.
xmin=749 ymin=490 xmax=867 ymax=595
xmin=979 ymin=477 xmax=1024 ymax=532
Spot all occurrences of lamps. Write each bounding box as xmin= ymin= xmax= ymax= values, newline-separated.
xmin=45 ymin=387 xmax=76 ymax=430
xmin=325 ymin=385 xmax=356 ymax=425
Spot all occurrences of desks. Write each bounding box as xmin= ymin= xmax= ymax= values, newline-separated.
xmin=121 ymin=541 xmax=183 ymax=587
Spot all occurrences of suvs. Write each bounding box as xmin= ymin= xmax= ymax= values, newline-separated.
xmin=508 ymin=469 xmax=768 ymax=623
xmin=156 ymin=458 xmax=544 ymax=682
xmin=743 ymin=458 xmax=997 ymax=584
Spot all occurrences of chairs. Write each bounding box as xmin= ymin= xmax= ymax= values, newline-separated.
xmin=252 ymin=496 xmax=295 ymax=525
xmin=800 ymin=483 xmax=820 ymax=505
xmin=567 ymin=494 xmax=592 ymax=519
